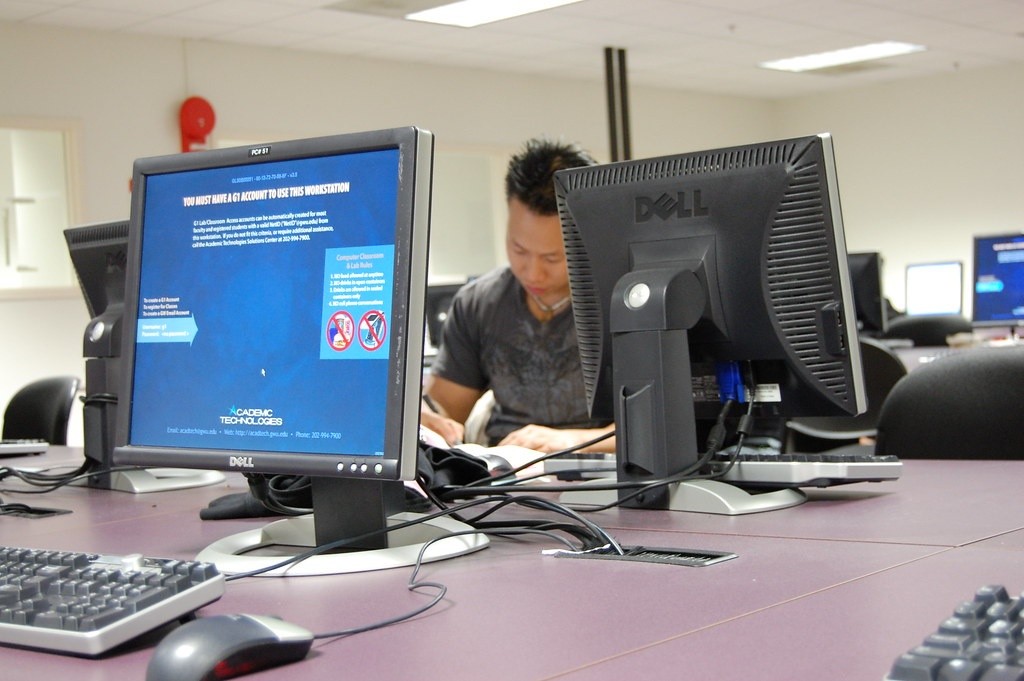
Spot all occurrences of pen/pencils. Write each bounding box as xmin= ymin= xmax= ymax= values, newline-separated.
xmin=423 ymin=394 xmax=439 ymax=414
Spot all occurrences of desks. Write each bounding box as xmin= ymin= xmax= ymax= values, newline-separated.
xmin=1 ymin=444 xmax=1023 ymax=681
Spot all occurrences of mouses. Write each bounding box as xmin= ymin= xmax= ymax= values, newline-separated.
xmin=477 ymin=454 xmax=515 ymax=482
xmin=144 ymin=613 xmax=312 ymax=681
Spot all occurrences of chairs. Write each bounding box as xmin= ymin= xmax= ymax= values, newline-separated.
xmin=1 ymin=376 xmax=79 ymax=445
xmin=786 ymin=313 xmax=1024 ymax=459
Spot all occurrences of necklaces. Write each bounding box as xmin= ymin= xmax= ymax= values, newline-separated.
xmin=531 ymin=294 xmax=572 ymax=336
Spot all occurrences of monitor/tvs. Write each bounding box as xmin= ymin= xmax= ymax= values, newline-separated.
xmin=63 ymin=220 xmax=226 ymax=493
xmin=555 ymin=133 xmax=870 ymax=517
xmin=849 ymin=232 xmax=1024 ymax=339
xmin=115 ymin=126 xmax=491 ymax=578
xmin=424 ymin=282 xmax=469 ymax=348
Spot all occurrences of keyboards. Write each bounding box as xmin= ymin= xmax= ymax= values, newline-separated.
xmin=0 ymin=547 xmax=224 ymax=658
xmin=544 ymin=451 xmax=904 ymax=492
xmin=0 ymin=439 xmax=49 ymax=457
xmin=887 ymin=584 xmax=1024 ymax=681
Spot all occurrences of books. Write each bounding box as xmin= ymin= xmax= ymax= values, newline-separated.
xmin=420 ymin=428 xmax=544 ymax=483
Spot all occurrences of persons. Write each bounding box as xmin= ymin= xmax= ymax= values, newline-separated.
xmin=422 ymin=142 xmax=620 ymax=454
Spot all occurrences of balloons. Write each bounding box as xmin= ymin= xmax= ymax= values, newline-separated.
xmin=178 ymin=97 xmax=215 ymax=139
